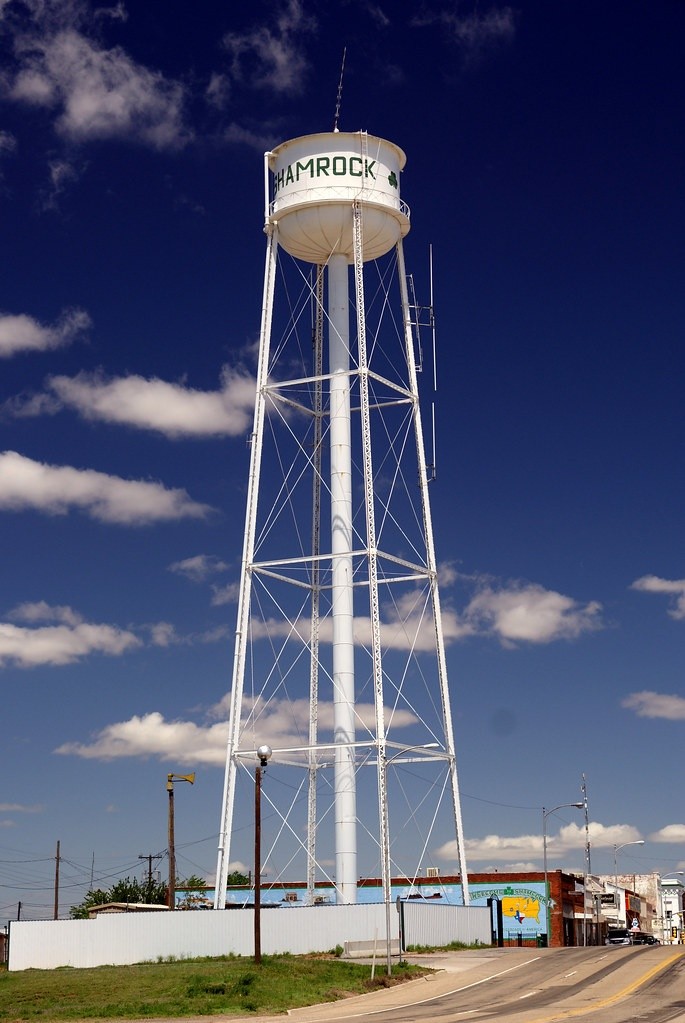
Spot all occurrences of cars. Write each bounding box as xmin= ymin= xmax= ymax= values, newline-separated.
xmin=603 ymin=929 xmax=660 ymax=947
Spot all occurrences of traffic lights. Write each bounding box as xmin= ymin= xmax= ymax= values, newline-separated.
xmin=672 ymin=927 xmax=677 ymax=938
xmin=680 ymin=930 xmax=685 ymax=941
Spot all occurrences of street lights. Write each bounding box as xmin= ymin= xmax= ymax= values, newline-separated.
xmin=542 ymin=802 xmax=583 ymax=950
xmin=254 ymin=743 xmax=271 ymax=963
xmin=614 ymin=841 xmax=644 ymax=929
xmin=660 ymin=871 xmax=684 ymax=944
